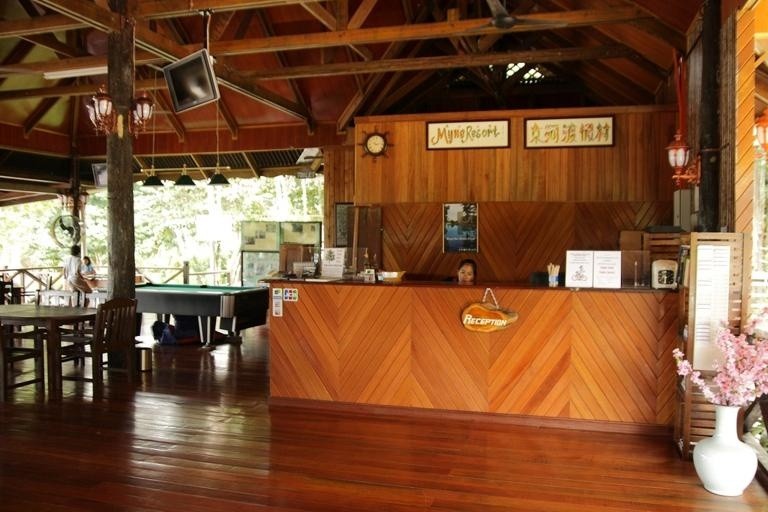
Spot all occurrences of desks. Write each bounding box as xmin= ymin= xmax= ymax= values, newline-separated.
xmin=133 ymin=284 xmax=269 ymax=347
xmin=0 ymin=302 xmax=99 ymax=391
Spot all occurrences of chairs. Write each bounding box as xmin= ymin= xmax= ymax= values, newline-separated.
xmin=1 ymin=323 xmax=42 ymax=392
xmin=57 ymin=299 xmax=138 ymax=384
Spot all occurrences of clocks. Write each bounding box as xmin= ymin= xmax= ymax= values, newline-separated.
xmin=356 ymin=125 xmax=393 ymax=165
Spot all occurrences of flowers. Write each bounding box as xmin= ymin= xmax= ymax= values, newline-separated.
xmin=672 ymin=325 xmax=763 ymax=406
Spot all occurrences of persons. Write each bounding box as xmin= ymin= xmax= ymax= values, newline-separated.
xmin=82 ymin=255 xmax=94 ymax=274
xmin=443 ymin=259 xmax=478 ymax=282
xmin=63 ymin=245 xmax=92 ymax=306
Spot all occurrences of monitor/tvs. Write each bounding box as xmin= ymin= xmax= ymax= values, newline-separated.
xmin=91 ymin=162 xmax=108 ymax=190
xmin=162 ymin=48 xmax=222 ymax=116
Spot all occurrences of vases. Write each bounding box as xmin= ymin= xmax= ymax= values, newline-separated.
xmin=691 ymin=402 xmax=758 ymax=496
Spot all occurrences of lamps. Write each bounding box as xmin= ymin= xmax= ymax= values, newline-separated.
xmin=664 ymin=127 xmax=691 ymax=186
xmin=143 ymin=104 xmax=229 ymax=191
